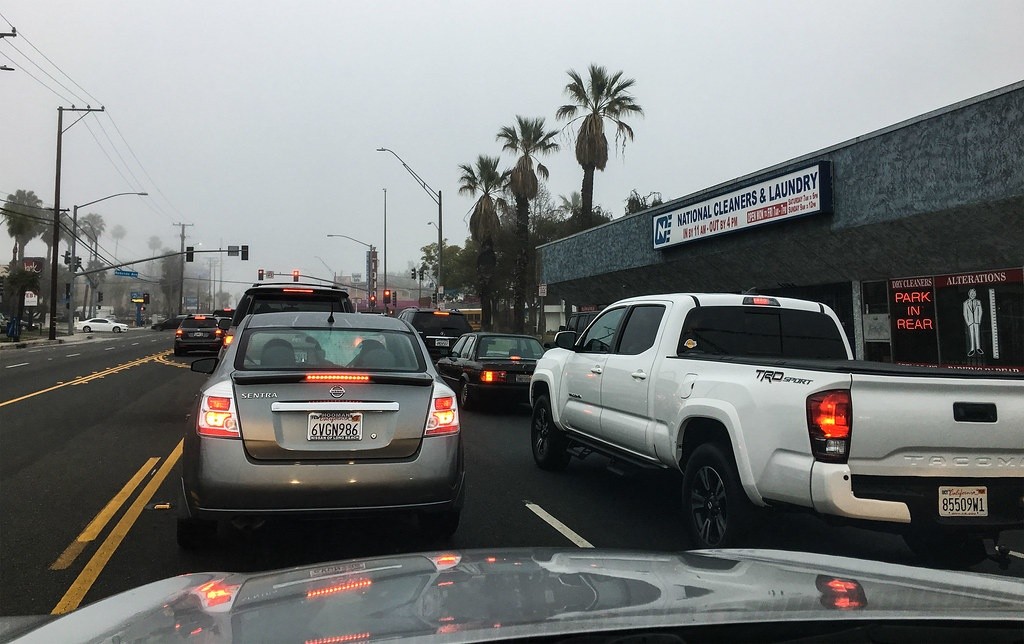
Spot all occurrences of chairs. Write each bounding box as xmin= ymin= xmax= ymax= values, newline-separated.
xmin=261 ymin=346 xmax=294 ymax=367
xmin=359 ymin=348 xmax=395 ymax=369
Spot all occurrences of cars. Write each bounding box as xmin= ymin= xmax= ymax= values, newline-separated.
xmin=434 ymin=332 xmax=546 ymax=411
xmin=212 ymin=308 xmax=236 ymax=334
xmin=173 ymin=313 xmax=226 ymax=357
xmin=151 ymin=314 xmax=188 ymax=331
xmin=0 ymin=315 xmax=36 ymax=334
xmin=190 ymin=311 xmax=461 ymax=552
xmin=77 ymin=317 xmax=129 ymax=333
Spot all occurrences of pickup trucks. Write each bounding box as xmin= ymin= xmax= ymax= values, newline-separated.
xmin=529 ymin=291 xmax=1024 ymax=563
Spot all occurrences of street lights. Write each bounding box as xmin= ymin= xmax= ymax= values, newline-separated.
xmin=67 ymin=192 xmax=150 ymax=333
xmin=376 ymin=148 xmax=442 ymax=308
xmin=327 ymin=234 xmax=377 ymax=312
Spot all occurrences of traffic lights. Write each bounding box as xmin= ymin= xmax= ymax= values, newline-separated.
xmin=392 ymin=292 xmax=397 ymax=307
xmin=419 ymin=270 xmax=423 ymax=281
xmin=258 ymin=270 xmax=263 ymax=280
xmin=64 ymin=251 xmax=71 ymax=265
xmin=143 ymin=294 xmax=150 ymax=305
xmin=293 ymin=271 xmax=298 ymax=281
xmin=140 ymin=307 xmax=145 ymax=310
xmin=411 ymin=268 xmax=416 ymax=280
xmin=383 ymin=290 xmax=391 ymax=304
xmin=370 ymin=296 xmax=376 ymax=307
xmin=77 ymin=257 xmax=82 ymax=268
xmin=98 ymin=291 xmax=104 ymax=302
xmin=98 ymin=305 xmax=101 ymax=309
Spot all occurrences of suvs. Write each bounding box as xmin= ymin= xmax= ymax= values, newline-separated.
xmin=217 ymin=282 xmax=356 ymax=361
xmin=398 ymin=306 xmax=475 ymax=367
xmin=557 ymin=311 xmax=601 ymax=347
